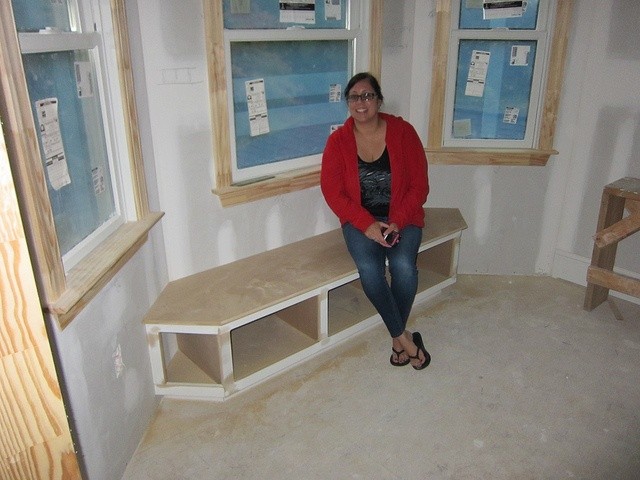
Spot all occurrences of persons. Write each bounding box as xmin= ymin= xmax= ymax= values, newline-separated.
xmin=320 ymin=71 xmax=430 ymax=370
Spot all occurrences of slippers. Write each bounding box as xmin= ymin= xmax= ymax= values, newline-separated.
xmin=409 ymin=332 xmax=431 ymax=370
xmin=390 ymin=347 xmax=411 ymax=366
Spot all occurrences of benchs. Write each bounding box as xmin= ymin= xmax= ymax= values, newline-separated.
xmin=142 ymin=206 xmax=468 ymax=401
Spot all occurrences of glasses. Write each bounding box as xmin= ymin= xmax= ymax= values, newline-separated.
xmin=347 ymin=92 xmax=375 ymax=102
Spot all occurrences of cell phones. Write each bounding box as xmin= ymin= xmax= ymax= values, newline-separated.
xmin=384 ymin=230 xmax=400 ymax=246
